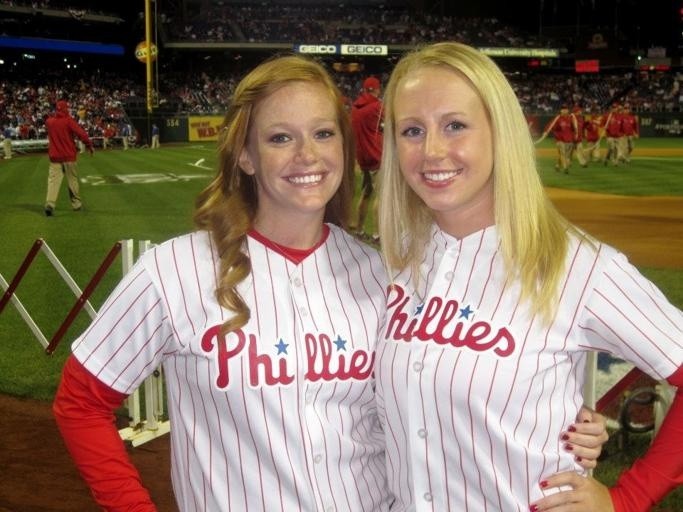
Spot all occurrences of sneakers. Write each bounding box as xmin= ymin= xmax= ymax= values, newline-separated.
xmin=354 ymin=230 xmax=380 ymax=248
xmin=44 ymin=206 xmax=81 ymax=217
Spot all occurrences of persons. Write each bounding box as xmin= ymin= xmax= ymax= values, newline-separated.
xmin=179 ymin=1 xmax=557 ymax=48
xmin=1 ymin=67 xmax=167 ymax=159
xmin=53 ymin=57 xmax=401 ymax=509
xmin=373 ymin=42 xmax=682 ymax=512
xmin=1 ymin=1 xmax=95 ymax=15
xmin=502 ymin=28 xmax=682 ymax=174
xmin=354 ymin=77 xmax=384 ymax=244
xmin=154 ymin=63 xmax=239 ymax=114
xmin=44 ymin=101 xmax=94 ymax=215
xmin=320 ymin=58 xmax=395 ymax=103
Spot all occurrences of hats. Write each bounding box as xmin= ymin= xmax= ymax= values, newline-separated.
xmin=364 ymin=76 xmax=381 ymax=91
xmin=561 ymin=102 xmax=631 ymax=113
xmin=54 ymin=100 xmax=69 ymax=110
